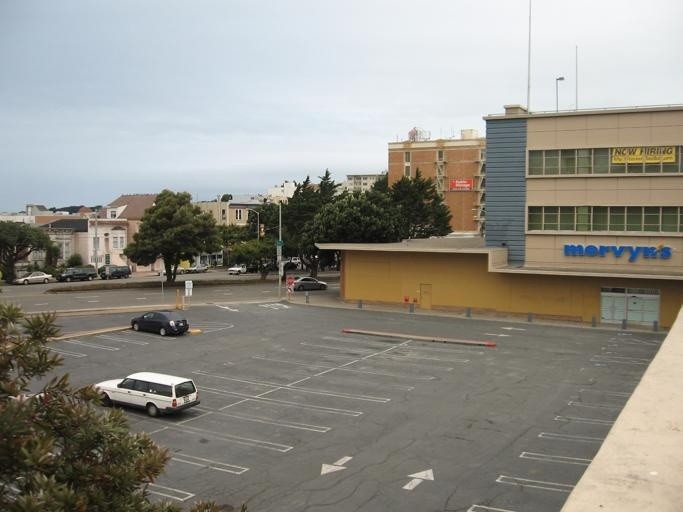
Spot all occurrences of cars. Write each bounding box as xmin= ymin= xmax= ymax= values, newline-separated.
xmin=164 ymin=262 xmax=208 ymax=275
xmin=228 ymin=263 xmax=258 ymax=275
xmin=290 ymin=257 xmax=301 ymax=266
xmin=293 ymin=277 xmax=328 ymax=290
xmin=99 ymin=268 xmax=132 ymax=280
xmin=131 ymin=311 xmax=190 ymax=336
xmin=12 ymin=271 xmax=53 ymax=285
xmin=94 ymin=371 xmax=201 ymax=417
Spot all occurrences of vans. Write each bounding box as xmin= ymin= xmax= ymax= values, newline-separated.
xmin=56 ymin=267 xmax=98 ymax=282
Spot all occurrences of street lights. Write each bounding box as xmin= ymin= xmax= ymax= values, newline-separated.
xmin=246 ymin=208 xmax=260 ymax=241
xmin=556 ymin=76 xmax=565 ymax=111
